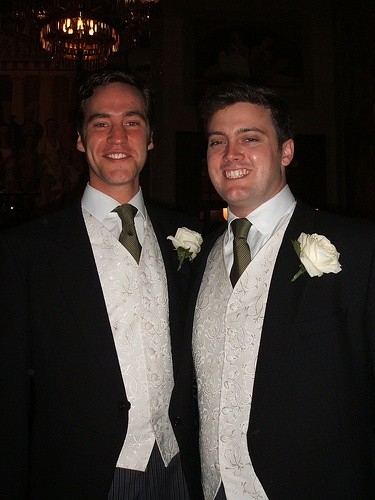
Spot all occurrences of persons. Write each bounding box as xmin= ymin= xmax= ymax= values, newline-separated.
xmin=173 ymin=81 xmax=375 ymax=500
xmin=0 ymin=69 xmax=215 ymax=500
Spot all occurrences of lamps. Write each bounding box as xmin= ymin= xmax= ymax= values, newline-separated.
xmin=14 ymin=0 xmax=158 ymax=64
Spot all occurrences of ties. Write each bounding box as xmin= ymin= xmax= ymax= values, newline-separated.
xmin=230 ymin=218 xmax=251 ymax=290
xmin=110 ymin=204 xmax=142 ymax=265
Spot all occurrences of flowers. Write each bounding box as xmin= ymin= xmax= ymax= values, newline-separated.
xmin=291 ymin=232 xmax=342 ymax=282
xmin=166 ymin=226 xmax=203 ymax=271
